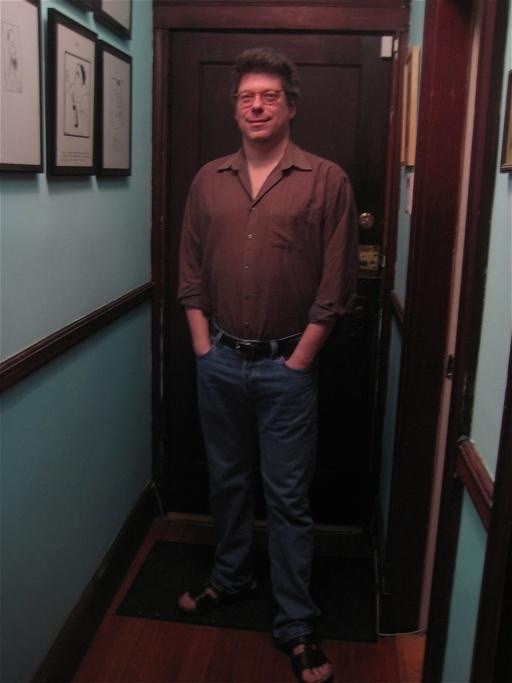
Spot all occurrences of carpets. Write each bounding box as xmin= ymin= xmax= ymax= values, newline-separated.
xmin=115 ymin=535 xmax=383 ymax=647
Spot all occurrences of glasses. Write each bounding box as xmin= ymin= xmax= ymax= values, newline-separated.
xmin=233 ymin=90 xmax=287 ymax=107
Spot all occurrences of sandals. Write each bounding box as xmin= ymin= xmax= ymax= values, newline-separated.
xmin=175 ymin=580 xmax=257 ymax=614
xmin=274 ymin=635 xmax=334 ymax=682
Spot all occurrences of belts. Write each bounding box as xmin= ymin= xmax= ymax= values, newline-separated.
xmin=210 ymin=320 xmax=302 ymax=353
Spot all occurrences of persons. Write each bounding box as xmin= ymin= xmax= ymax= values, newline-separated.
xmin=147 ymin=46 xmax=364 ymax=683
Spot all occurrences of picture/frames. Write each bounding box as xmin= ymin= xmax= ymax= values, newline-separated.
xmin=397 ymin=42 xmax=422 ymax=168
xmin=93 ymin=0 xmax=134 ymax=40
xmin=496 ymin=65 xmax=512 ymax=173
xmin=96 ymin=38 xmax=134 ymax=179
xmin=1 ymin=0 xmax=46 ymax=174
xmin=43 ymin=6 xmax=100 ymax=179
